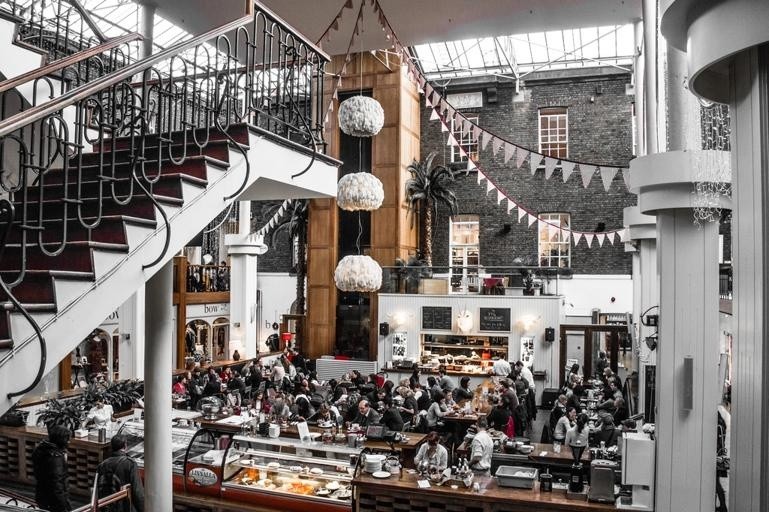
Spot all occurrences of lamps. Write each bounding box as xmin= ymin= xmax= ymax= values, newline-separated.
xmin=333 ymin=0 xmax=386 ymax=293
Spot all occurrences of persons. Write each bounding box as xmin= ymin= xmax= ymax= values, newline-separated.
xmin=206 ymin=262 xmax=216 ymax=290
xmin=32 ymin=425 xmax=73 ymax=512
xmin=174 ymin=334 xmax=455 ymax=433
xmin=79 ymin=395 xmax=122 ymax=429
xmin=91 ymin=434 xmax=145 ymax=512
xmin=414 ymin=349 xmax=632 ymax=476
xmin=187 ymin=261 xmax=204 ymax=292
xmin=217 ymin=260 xmax=229 ymax=291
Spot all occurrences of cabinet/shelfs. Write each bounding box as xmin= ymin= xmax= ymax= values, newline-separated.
xmin=421 ymin=333 xmax=508 ymax=364
xmin=126 ymin=429 xmax=370 ymax=512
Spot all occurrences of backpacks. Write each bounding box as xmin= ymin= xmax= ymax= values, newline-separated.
xmin=98 ymin=457 xmax=126 ymax=511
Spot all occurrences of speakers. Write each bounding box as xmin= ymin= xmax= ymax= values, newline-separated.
xmin=545 ymin=328 xmax=554 ymax=341
xmin=380 ymin=323 xmax=389 ymax=336
xmin=503 ymin=223 xmax=511 ymax=234
xmin=597 ymin=221 xmax=605 ymax=232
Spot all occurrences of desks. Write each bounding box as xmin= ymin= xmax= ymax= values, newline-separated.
xmin=441 ymin=397 xmax=482 ymax=439
xmin=190 ymin=410 xmax=426 ymax=456
xmin=457 ymin=432 xmax=599 ymax=470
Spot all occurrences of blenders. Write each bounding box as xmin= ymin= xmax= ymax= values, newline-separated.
xmin=568 ymin=438 xmax=586 ymax=493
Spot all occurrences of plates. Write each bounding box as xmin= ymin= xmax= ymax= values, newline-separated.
xmin=516 ymin=442 xmax=531 ymax=454
xmin=364 ymin=455 xmax=390 ymax=479
xmin=317 ymin=424 xmax=332 ymax=428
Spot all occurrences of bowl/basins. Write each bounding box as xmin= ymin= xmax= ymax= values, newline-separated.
xmin=259 ymin=421 xmax=280 ymax=439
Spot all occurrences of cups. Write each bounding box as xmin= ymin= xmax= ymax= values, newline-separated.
xmin=99 ymin=428 xmax=106 ymax=442
xmin=347 ymin=433 xmax=356 ymax=447
xmin=554 ymin=441 xmax=561 ymax=454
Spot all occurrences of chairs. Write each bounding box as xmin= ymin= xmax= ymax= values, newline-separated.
xmin=230 ymin=382 xmax=266 ymax=400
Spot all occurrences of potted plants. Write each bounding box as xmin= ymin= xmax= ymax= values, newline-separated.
xmin=0 ymin=375 xmax=144 ymax=435
